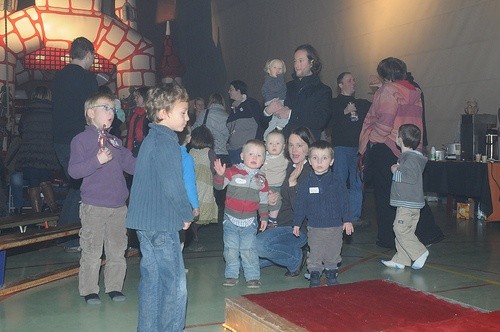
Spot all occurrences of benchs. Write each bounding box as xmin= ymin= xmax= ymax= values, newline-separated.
xmin=0 ymin=207 xmax=141 ymax=296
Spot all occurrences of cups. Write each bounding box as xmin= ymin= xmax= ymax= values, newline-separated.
xmin=436 ymin=151 xmax=442 ymax=161
xmin=475 ymin=155 xmax=481 ymax=162
xmin=482 ymin=156 xmax=487 ymax=163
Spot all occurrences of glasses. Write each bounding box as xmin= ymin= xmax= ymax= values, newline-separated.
xmin=90 ymin=104 xmax=117 ymax=114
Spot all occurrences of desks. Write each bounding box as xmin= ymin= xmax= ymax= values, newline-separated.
xmin=422 ymin=159 xmax=500 ymax=223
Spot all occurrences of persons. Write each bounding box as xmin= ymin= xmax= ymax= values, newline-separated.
xmin=250 ymin=126 xmax=316 ymax=279
xmin=381 ymin=124 xmax=429 ymax=270
xmin=125 ymin=80 xmax=195 ymax=332
xmin=291 ymin=139 xmax=354 ymax=290
xmin=213 ymin=138 xmax=270 ymax=289
xmin=48 ymin=36 xmax=431 ymax=305
xmin=68 ymin=91 xmax=138 ymax=304
xmin=0 ymin=86 xmax=58 ymax=218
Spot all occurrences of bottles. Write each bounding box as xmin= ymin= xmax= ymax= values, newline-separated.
xmin=430 ymin=146 xmax=435 ymax=160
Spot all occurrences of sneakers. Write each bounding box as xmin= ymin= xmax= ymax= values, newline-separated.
xmin=310 ymin=271 xmax=321 ymax=288
xmin=325 ymin=270 xmax=339 ymax=285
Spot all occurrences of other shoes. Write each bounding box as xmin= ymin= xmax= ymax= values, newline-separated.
xmin=223 ymin=278 xmax=239 ymax=286
xmin=285 ymin=250 xmax=306 ymax=278
xmin=305 ymin=269 xmax=311 ymax=279
xmin=247 ymin=280 xmax=261 ymax=288
xmin=376 ymin=241 xmax=397 ymax=250
xmin=421 ymin=232 xmax=445 ymax=248
xmin=353 ymin=220 xmax=371 ymax=227
